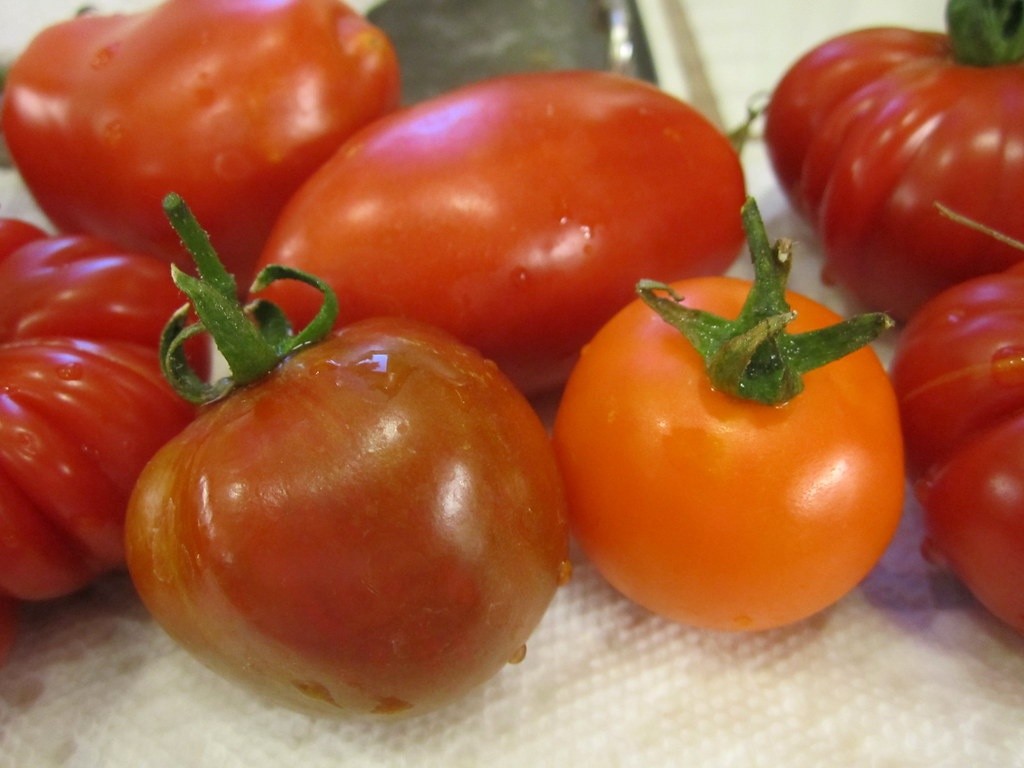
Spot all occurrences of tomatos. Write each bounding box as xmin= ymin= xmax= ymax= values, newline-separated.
xmin=1 ymin=0 xmax=1024 ymax=718
